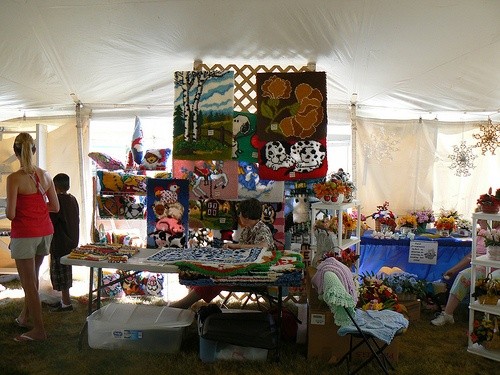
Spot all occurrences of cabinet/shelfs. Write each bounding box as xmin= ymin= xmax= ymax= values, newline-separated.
xmin=310 ymin=202 xmax=361 ymax=274
xmin=467 ymin=211 xmax=500 ymax=362
xmin=88 ymin=174 xmax=190 ymax=300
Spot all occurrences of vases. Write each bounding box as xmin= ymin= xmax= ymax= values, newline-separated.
xmin=487 ymin=245 xmax=500 ymax=261
xmin=481 ymin=199 xmax=500 ymax=214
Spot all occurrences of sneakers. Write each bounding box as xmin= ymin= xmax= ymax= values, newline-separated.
xmin=430 ymin=311 xmax=455 ymax=327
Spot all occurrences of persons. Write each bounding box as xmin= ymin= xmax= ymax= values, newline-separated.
xmin=166 ymin=198 xmax=275 ymax=310
xmin=5 ymin=132 xmax=60 ymax=345
xmin=431 ymin=207 xmax=500 ymax=326
xmin=47 ymin=173 xmax=80 ymax=314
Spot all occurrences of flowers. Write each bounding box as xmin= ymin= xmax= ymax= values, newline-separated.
xmin=315 ymin=168 xmax=500 ymax=346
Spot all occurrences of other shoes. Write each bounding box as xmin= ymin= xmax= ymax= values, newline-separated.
xmin=48 ymin=300 xmax=73 ymax=313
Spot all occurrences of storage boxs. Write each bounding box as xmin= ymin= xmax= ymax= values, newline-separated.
xmin=87 ymin=301 xmax=195 ymax=353
xmin=309 ymin=309 xmax=351 ymax=363
xmin=352 ymin=329 xmax=400 ymax=365
xmin=391 ymin=295 xmax=421 ymax=322
xmin=197 ymin=303 xmax=275 ymax=362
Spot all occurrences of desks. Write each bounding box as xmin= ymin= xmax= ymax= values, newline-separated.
xmin=60 ymin=243 xmax=305 ymax=361
xmin=350 ymin=228 xmax=473 ymax=301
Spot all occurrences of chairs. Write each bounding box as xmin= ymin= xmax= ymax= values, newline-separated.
xmin=314 ymin=256 xmax=409 ymax=375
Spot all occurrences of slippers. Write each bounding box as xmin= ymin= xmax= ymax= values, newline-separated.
xmin=14 ymin=317 xmax=29 ymax=328
xmin=13 ymin=333 xmax=48 ymax=343
